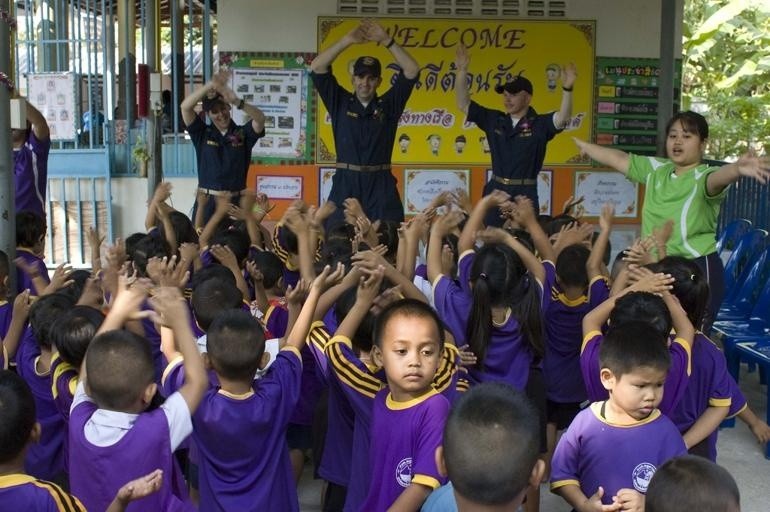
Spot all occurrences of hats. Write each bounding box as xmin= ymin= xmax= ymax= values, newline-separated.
xmin=203 ymin=91 xmax=224 ymax=111
xmin=496 ymin=76 xmax=532 ymax=95
xmin=354 ymin=57 xmax=380 ymax=76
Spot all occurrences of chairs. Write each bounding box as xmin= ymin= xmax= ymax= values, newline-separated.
xmin=692 ymin=216 xmax=770 ymax=432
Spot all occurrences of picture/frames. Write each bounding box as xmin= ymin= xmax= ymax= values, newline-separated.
xmin=24 ymin=72 xmax=81 ymax=143
xmin=402 ymin=167 xmax=471 ymax=217
xmin=254 ymin=175 xmax=304 ymax=201
xmin=486 ymin=168 xmax=554 ymax=217
xmin=572 ymin=169 xmax=640 ymax=220
xmin=318 ymin=166 xmax=338 ymax=209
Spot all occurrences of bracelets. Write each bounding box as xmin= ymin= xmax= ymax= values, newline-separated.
xmin=385 ymin=38 xmax=395 ymax=50
xmin=562 ymin=85 xmax=574 ymax=92
xmin=236 ymin=99 xmax=244 ymax=110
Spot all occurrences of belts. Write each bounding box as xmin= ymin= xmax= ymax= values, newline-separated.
xmin=492 ymin=175 xmax=535 ymax=185
xmin=336 ymin=163 xmax=391 ymax=171
xmin=200 ymin=188 xmax=246 ymax=197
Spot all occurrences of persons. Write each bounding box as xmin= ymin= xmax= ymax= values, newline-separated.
xmin=453 ymin=41 xmax=579 ymax=228
xmin=0 ymin=69 xmax=52 ymax=215
xmin=568 ymin=109 xmax=770 ymax=338
xmin=179 ymin=67 xmax=267 ymax=231
xmin=158 ymin=88 xmax=185 ymax=135
xmin=308 ymin=15 xmax=423 ymax=234
xmin=1 ymin=181 xmax=770 ymax=511
